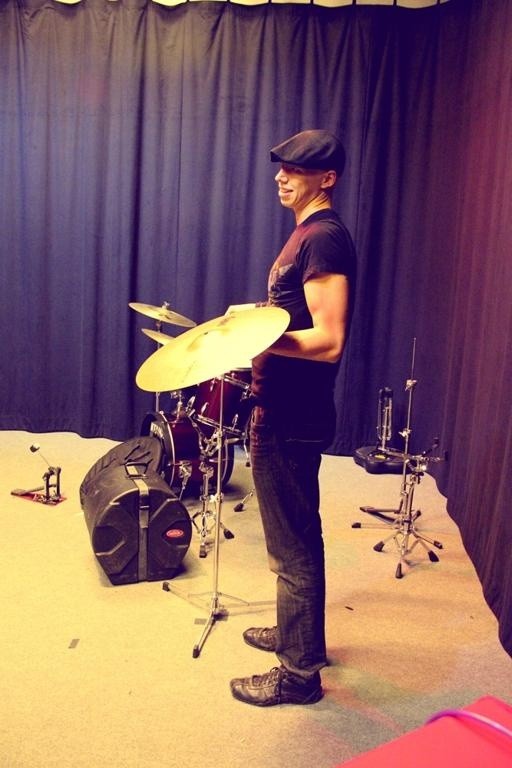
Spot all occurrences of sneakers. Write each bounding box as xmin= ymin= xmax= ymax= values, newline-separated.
xmin=230 ymin=666 xmax=323 ymax=707
xmin=243 ymin=626 xmax=277 ymax=652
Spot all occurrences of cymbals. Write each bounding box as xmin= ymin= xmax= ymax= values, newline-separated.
xmin=142 ymin=328 xmax=175 ymax=344
xmin=135 ymin=306 xmax=290 ymax=392
xmin=129 ymin=302 xmax=196 ymax=327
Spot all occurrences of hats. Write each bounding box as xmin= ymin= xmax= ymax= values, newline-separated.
xmin=270 ymin=129 xmax=346 ymax=177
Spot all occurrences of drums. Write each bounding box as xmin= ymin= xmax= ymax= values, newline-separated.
xmin=188 ymin=375 xmax=255 ymax=439
xmin=222 ymin=360 xmax=253 ymax=386
xmin=141 ymin=411 xmax=234 ymax=491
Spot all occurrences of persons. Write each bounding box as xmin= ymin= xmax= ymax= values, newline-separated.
xmin=224 ymin=126 xmax=358 ymax=707
